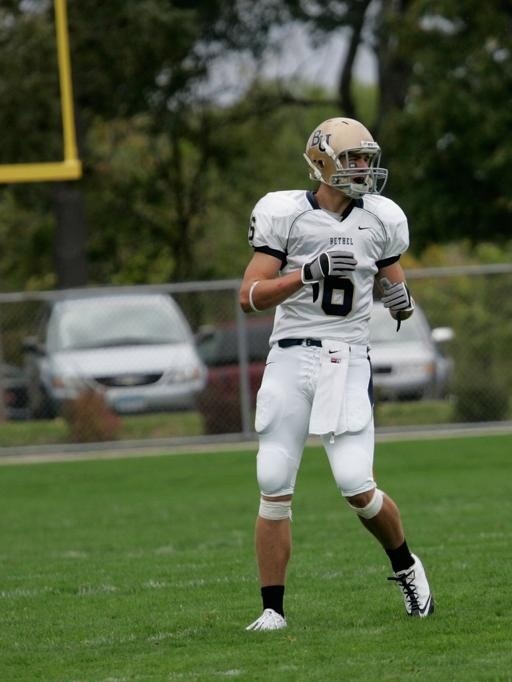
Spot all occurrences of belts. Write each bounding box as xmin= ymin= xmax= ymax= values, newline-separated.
xmin=278 ymin=339 xmax=321 ymax=348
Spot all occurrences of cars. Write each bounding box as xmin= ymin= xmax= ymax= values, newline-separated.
xmin=0 ymin=360 xmax=31 ymax=420
xmin=24 ymin=290 xmax=214 ymax=418
xmin=360 ymin=298 xmax=454 ymax=404
xmin=195 ymin=315 xmax=272 ymax=431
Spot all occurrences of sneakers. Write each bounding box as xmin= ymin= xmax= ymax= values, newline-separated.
xmin=386 ymin=552 xmax=434 ymax=618
xmin=245 ymin=609 xmax=287 ymax=632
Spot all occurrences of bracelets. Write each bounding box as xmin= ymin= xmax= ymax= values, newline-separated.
xmin=249 ymin=281 xmax=263 ymax=312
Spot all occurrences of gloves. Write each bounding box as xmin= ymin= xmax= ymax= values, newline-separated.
xmin=300 ymin=250 xmax=357 ymax=284
xmin=380 ymin=277 xmax=416 ymax=331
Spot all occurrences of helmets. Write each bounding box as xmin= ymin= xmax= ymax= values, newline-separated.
xmin=304 ymin=118 xmax=388 ymax=200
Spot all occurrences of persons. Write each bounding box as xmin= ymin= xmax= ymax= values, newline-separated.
xmin=234 ymin=116 xmax=434 ymax=632
